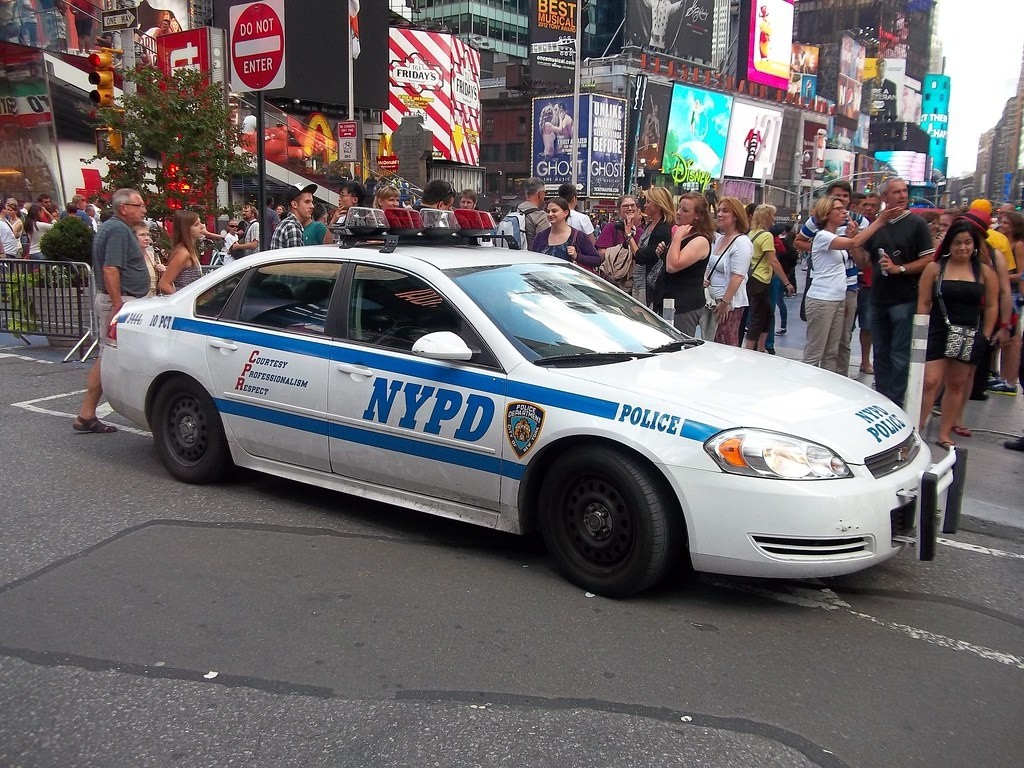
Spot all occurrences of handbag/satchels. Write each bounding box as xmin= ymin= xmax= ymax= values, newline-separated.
xmin=800 ymin=278 xmax=813 ymax=322
xmin=647 ymin=258 xmax=665 ymax=291
xmin=21 ymin=243 xmax=31 ymax=259
xmin=229 ymin=234 xmax=245 ymax=259
xmin=571 ymin=230 xmax=599 ymax=274
xmin=944 ymin=325 xmax=979 ymax=364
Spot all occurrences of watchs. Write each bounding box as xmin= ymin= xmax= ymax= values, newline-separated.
xmin=899 ymin=264 xmax=905 ymax=273
xmin=1001 ymin=320 xmax=1012 ymax=330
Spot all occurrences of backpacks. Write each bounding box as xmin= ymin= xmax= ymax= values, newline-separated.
xmin=495 ymin=205 xmax=547 ymax=251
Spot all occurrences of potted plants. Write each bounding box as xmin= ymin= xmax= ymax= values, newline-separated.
xmin=28 ymin=215 xmax=97 ymax=347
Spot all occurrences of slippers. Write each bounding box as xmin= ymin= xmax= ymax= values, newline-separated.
xmin=935 ymin=439 xmax=959 ymax=450
xmin=951 ymin=426 xmax=972 ymax=437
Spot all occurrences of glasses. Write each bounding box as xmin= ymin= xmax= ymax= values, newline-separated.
xmin=124 ymin=203 xmax=146 ymax=208
xmin=825 ymin=206 xmax=844 ymax=216
xmin=538 ymin=190 xmax=548 ymax=195
xmin=339 ymin=193 xmax=350 ymax=198
xmin=228 ymin=224 xmax=238 ymax=229
xmin=622 ymin=204 xmax=635 ymax=209
xmin=439 ymin=188 xmax=456 ymax=204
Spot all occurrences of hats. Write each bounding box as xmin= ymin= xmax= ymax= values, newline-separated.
xmin=971 ymin=199 xmax=992 ymax=215
xmin=89 ymin=196 xmax=99 ymax=201
xmin=956 ymin=209 xmax=991 ymax=239
xmin=287 ymin=182 xmax=318 ymax=204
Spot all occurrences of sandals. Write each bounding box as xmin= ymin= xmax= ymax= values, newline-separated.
xmin=72 ymin=416 xmax=119 ymax=433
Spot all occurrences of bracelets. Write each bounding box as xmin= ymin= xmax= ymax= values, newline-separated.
xmin=626 ymin=232 xmax=633 ymax=242
xmin=720 ymin=299 xmax=728 ymax=305
xmin=784 ymin=281 xmax=791 ymax=287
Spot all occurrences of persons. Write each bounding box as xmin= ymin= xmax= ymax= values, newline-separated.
xmin=859 ymin=59 xmax=897 ymax=121
xmin=1 ymin=177 xmax=1024 ymax=450
xmin=538 ymin=102 xmax=573 ymax=156
xmin=0 ymin=0 xmax=38 ymax=47
xmin=153 ymin=19 xmax=172 ymax=41
xmin=96 ymin=31 xmax=112 ymax=48
xmin=69 ymin=0 xmax=97 ymax=55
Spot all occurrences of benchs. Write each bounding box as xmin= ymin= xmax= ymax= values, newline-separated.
xmin=205 ymin=278 xmax=337 ymax=323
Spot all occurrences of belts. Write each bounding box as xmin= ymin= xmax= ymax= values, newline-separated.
xmin=102 ymin=291 xmax=132 ymax=296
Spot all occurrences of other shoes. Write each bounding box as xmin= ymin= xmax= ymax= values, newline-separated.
xmin=768 ymin=347 xmax=776 ymax=355
xmin=860 ymin=363 xmax=874 ymax=374
xmin=774 ymin=328 xmax=787 ymax=335
xmin=1004 ymin=436 xmax=1024 ymax=451
xmin=931 ymin=371 xmax=1018 ymax=415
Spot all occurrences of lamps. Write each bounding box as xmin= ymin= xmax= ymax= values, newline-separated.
xmin=478 ymin=42 xmax=489 ymax=46
xmin=472 ymin=36 xmax=483 ymax=42
